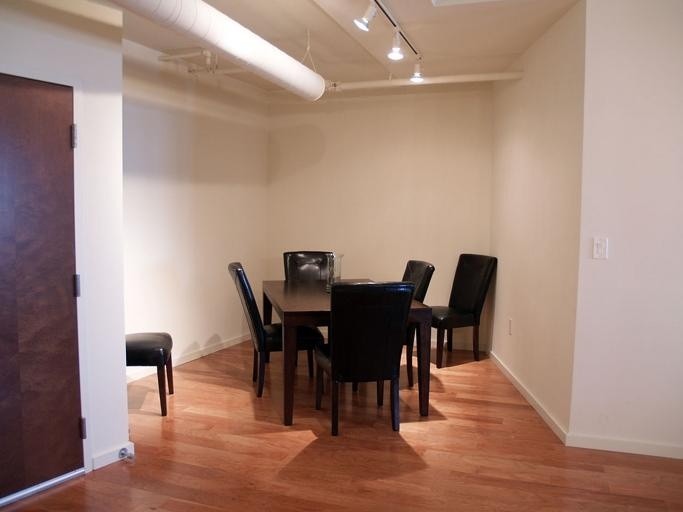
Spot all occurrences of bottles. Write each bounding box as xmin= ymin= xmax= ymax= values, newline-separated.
xmin=324 ymin=248 xmax=344 ymax=293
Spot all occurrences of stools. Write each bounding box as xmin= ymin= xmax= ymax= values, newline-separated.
xmin=127 ymin=332 xmax=174 ymax=417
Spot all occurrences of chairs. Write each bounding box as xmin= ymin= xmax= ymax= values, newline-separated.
xmin=228 ymin=260 xmax=323 ymax=398
xmin=429 ymin=252 xmax=498 ymax=371
xmin=352 ymin=259 xmax=435 ymax=393
xmin=283 ymin=252 xmax=335 ymax=376
xmin=316 ymin=281 xmax=414 ymax=437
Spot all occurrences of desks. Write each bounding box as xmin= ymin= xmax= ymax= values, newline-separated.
xmin=263 ymin=278 xmax=433 ymax=425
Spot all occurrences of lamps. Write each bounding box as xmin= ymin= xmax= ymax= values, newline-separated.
xmin=353 ymin=2 xmax=424 ymax=84
